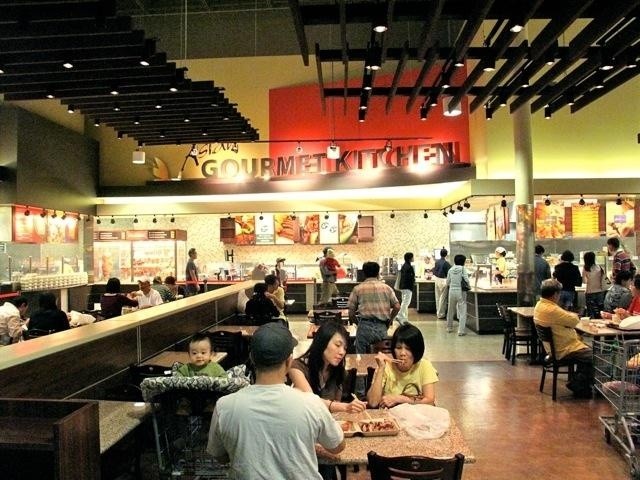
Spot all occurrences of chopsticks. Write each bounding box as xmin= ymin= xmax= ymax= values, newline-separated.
xmin=351 ymin=393 xmax=372 ymax=420
xmin=392 ymin=359 xmax=402 ymax=363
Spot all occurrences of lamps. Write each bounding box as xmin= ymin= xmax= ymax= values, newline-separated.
xmin=23 ymin=204 xmax=178 ymax=225
xmin=327 ymin=61 xmax=340 ymax=159
xmin=227 ymin=197 xmax=472 ymax=221
xmin=132 ymin=146 xmax=145 ymax=165
xmin=45 ymin=55 xmax=248 ymax=139
xmin=358 ymin=20 xmax=637 ymax=123
xmin=501 ymin=191 xmax=624 ymax=207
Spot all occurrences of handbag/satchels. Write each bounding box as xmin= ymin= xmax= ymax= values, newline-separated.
xmin=461 ymin=277 xmax=471 ymax=291
xmin=393 ymin=270 xmax=402 ymax=291
xmin=601 ymin=273 xmax=613 ymax=291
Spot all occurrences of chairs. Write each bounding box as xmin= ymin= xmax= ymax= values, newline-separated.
xmin=367 ymin=450 xmax=464 ymax=480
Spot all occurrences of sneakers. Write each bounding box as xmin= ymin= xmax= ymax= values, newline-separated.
xmin=459 ymin=332 xmax=467 ymax=336
xmin=565 ymin=380 xmax=594 ymax=399
xmin=395 ymin=315 xmax=404 ymax=326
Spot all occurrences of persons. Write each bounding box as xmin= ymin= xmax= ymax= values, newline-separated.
xmin=347 ymin=261 xmax=400 ymax=394
xmin=186 ymin=248 xmax=200 ymax=294
xmin=1 ymin=293 xmax=72 ymax=345
xmin=22 ymin=261 xmax=40 ymax=274
xmin=433 ymin=249 xmax=470 ymax=336
xmin=493 ymin=247 xmax=507 ymax=284
xmin=173 ymin=332 xmax=227 ymax=408
xmin=245 ymin=257 xmax=288 ymax=327
xmin=396 ymin=253 xmax=415 ymax=325
xmin=205 ymin=322 xmax=439 ymax=479
xmin=534 ymin=239 xmax=640 ymax=399
xmin=100 ymin=276 xmax=187 ymax=320
xmin=57 ymin=258 xmax=75 ymax=274
xmin=309 ymin=247 xmax=341 ymax=326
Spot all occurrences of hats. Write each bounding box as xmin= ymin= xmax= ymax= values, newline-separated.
xmin=137 ymin=274 xmax=151 ymax=284
xmin=327 ymin=249 xmax=336 ymax=259
xmin=276 ymin=257 xmax=285 ymax=262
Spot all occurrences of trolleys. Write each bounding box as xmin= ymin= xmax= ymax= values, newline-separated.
xmin=592 ymin=338 xmax=640 ymax=480
xmin=144 ymin=363 xmax=257 ymax=479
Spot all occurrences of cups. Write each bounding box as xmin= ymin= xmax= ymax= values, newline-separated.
xmin=134 ymin=402 xmax=145 ymax=418
xmin=580 ymin=317 xmax=590 ymax=326
